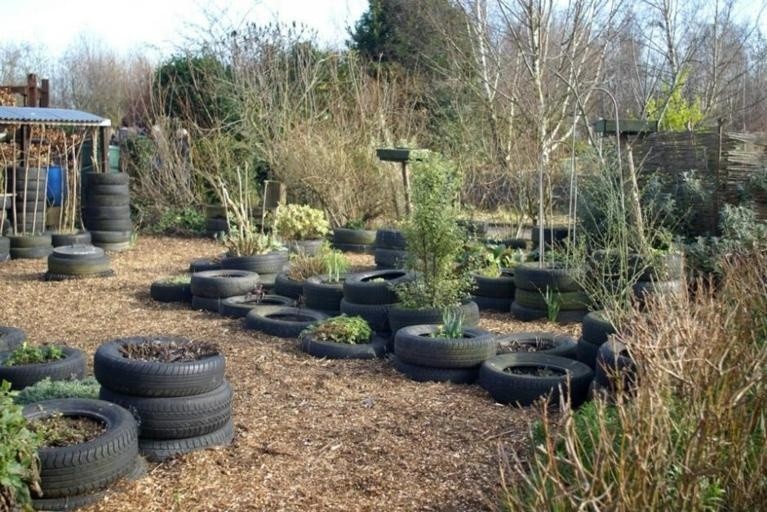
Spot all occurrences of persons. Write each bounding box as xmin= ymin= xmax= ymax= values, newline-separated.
xmin=111 ymin=115 xmax=190 ymax=148
